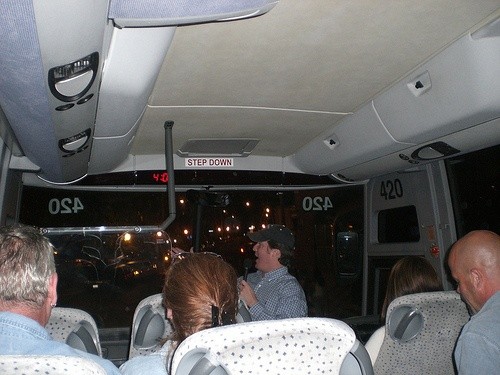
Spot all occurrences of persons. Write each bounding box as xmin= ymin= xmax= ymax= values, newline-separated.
xmin=447 ymin=230 xmax=500 ymax=375
xmin=0 ymin=222 xmax=122 ymax=375
xmin=364 ymin=256 xmax=444 ymax=369
xmin=118 ymin=252 xmax=239 ymax=375
xmin=167 ymin=224 xmax=308 ymax=325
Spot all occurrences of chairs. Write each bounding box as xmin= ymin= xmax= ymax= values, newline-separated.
xmin=51 ymin=307 xmax=102 ymax=358
xmin=129 ymin=294 xmax=174 ymax=358
xmin=373 ymin=291 xmax=470 ymax=375
xmin=167 ymin=317 xmax=374 ymax=375
xmin=0 ymin=356 xmax=108 ymax=375
xmin=46 ymin=317 xmax=98 ymax=355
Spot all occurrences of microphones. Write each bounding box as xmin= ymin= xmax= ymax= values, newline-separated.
xmin=242 ymin=258 xmax=253 ymax=281
